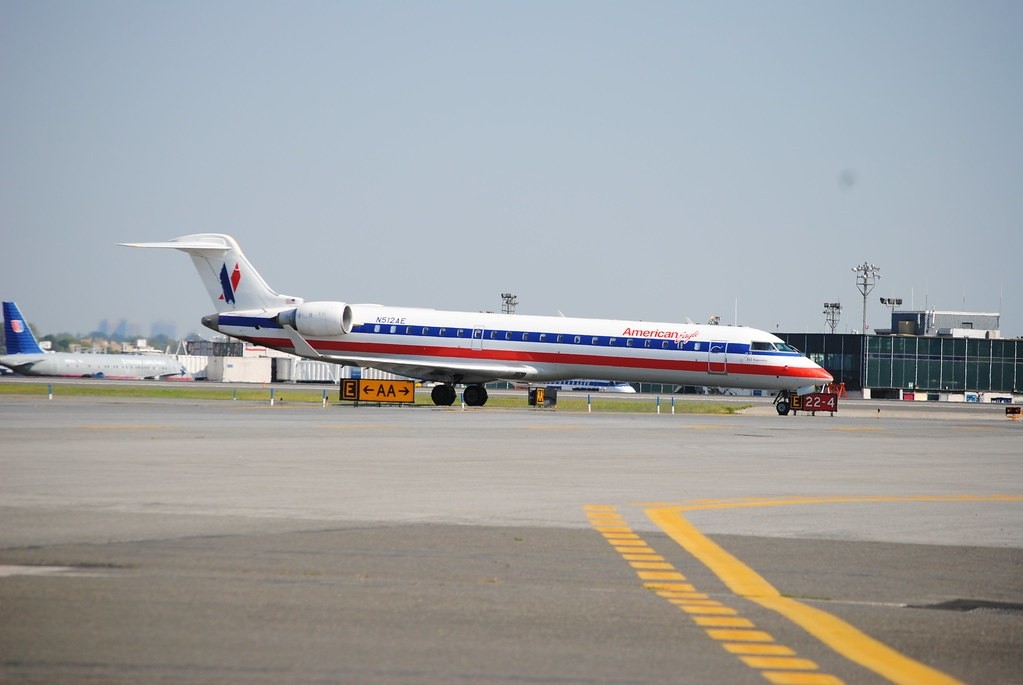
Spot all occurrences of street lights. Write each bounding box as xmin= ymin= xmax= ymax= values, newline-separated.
xmin=851 ymin=261 xmax=882 ymax=390
xmin=824 ymin=301 xmax=843 ymax=335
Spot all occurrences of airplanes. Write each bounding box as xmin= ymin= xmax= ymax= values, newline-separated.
xmin=118 ymin=232 xmax=835 ymax=418
xmin=509 ymin=378 xmax=638 ymax=394
xmin=0 ymin=300 xmax=195 ymax=381
